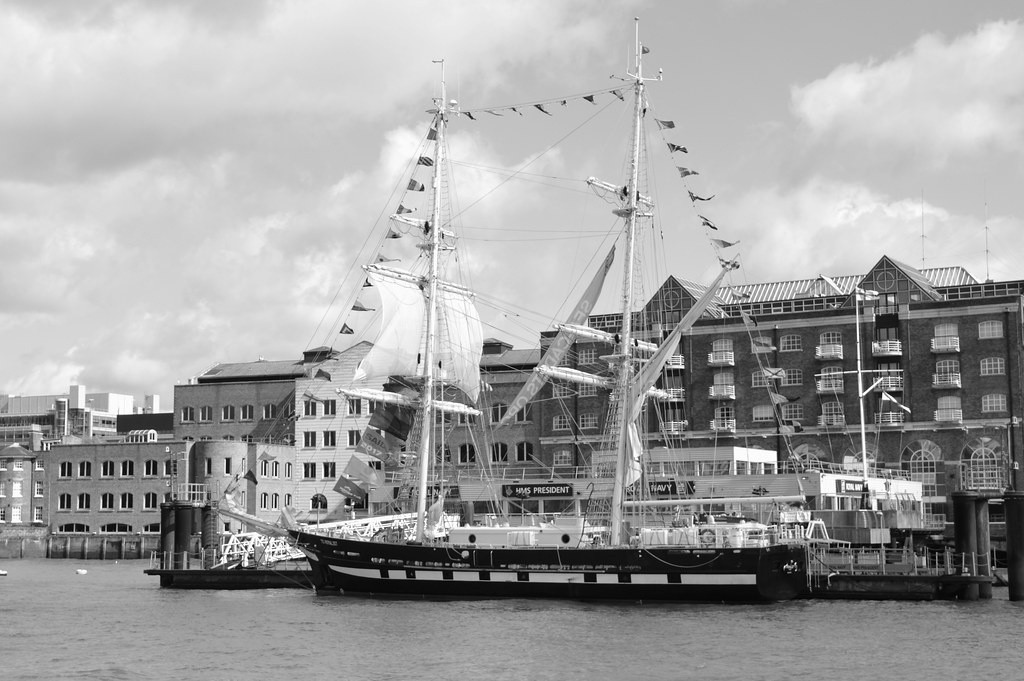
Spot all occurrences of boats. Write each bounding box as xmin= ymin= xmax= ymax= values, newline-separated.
xmin=139 ymin=13 xmax=955 ymax=599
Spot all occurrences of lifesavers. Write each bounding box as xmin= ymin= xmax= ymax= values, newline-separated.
xmin=700 ymin=527 xmax=716 ymax=547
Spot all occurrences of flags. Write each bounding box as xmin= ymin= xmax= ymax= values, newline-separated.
xmin=880 ymin=393 xmax=912 ymax=414
